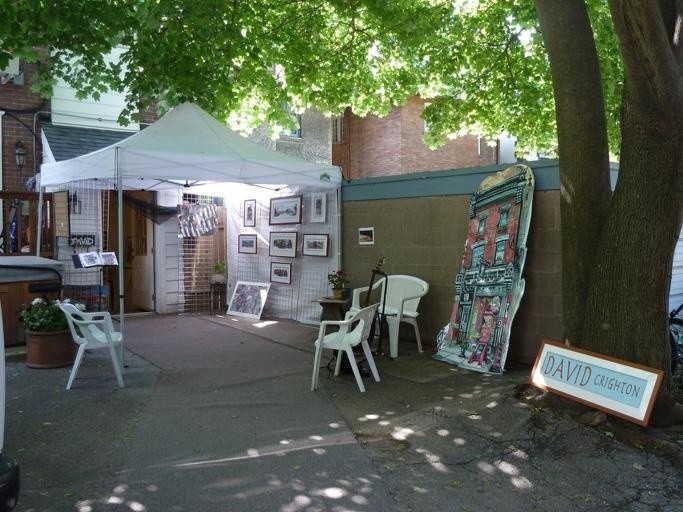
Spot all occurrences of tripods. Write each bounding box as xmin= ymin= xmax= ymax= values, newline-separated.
xmin=94 ymin=268 xmax=120 ymax=323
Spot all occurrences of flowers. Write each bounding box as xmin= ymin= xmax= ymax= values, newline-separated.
xmin=327 ymin=270 xmax=350 ymax=289
xmin=15 ymin=296 xmax=86 ymax=331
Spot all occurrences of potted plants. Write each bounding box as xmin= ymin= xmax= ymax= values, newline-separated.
xmin=210 ymin=262 xmax=226 ymax=284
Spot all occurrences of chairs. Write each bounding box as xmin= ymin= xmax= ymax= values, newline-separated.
xmin=310 ymin=274 xmax=429 ymax=393
xmin=58 ymin=302 xmax=126 ymax=389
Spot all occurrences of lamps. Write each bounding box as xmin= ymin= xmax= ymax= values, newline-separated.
xmin=14 ymin=142 xmax=25 ymax=171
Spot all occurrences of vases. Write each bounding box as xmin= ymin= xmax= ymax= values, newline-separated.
xmin=23 ymin=327 xmax=84 ymax=369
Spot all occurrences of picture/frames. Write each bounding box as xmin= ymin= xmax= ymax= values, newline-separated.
xmin=529 ymin=339 xmax=666 ymax=429
xmin=237 ymin=191 xmax=329 ymax=283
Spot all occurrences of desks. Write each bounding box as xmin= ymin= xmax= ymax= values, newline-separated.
xmin=210 ymin=284 xmax=226 ymax=315
